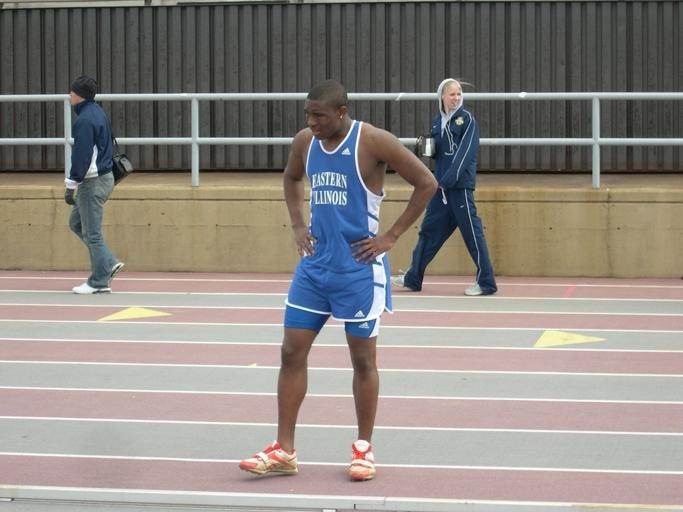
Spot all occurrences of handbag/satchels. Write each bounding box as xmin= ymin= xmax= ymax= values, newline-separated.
xmin=111 ymin=152 xmax=133 ymax=185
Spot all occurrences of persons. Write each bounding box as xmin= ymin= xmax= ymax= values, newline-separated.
xmin=390 ymin=78 xmax=497 ymax=296
xmin=64 ymin=74 xmax=125 ymax=294
xmin=239 ymin=79 xmax=439 ymax=481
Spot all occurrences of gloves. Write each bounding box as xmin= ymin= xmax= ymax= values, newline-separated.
xmin=64 ymin=177 xmax=83 ymax=206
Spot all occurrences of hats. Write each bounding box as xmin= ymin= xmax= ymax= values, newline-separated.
xmin=70 ymin=75 xmax=98 ymax=100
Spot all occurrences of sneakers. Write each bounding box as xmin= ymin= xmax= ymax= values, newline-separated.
xmin=389 ymin=269 xmax=422 ymax=291
xmin=72 ymin=278 xmax=112 ymax=295
xmin=110 ymin=262 xmax=124 ymax=281
xmin=464 ymin=281 xmax=498 ymax=296
xmin=238 ymin=439 xmax=298 ymax=477
xmin=349 ymin=439 xmax=375 ymax=480
